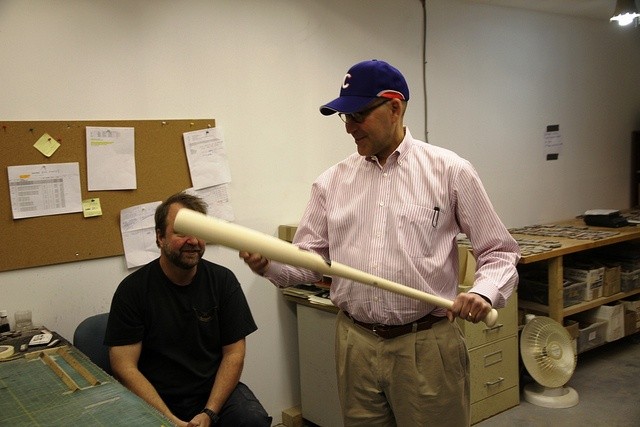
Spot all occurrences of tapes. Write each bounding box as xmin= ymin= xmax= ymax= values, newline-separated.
xmin=0 ymin=345 xmax=15 ymax=359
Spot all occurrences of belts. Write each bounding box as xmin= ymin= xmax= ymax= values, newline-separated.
xmin=342 ymin=311 xmax=447 ymax=339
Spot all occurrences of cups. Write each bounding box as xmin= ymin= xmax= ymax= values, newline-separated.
xmin=14 ymin=309 xmax=32 ymax=330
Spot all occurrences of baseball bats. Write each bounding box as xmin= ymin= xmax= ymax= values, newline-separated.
xmin=173 ymin=209 xmax=499 ymax=328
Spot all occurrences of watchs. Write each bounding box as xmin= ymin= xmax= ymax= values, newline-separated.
xmin=200 ymin=408 xmax=220 ymax=424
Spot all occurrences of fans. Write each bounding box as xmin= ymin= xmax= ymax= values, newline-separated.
xmin=518 ymin=316 xmax=581 ymax=408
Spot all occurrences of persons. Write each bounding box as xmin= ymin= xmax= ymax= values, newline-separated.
xmin=239 ymin=59 xmax=521 ymax=427
xmin=103 ymin=193 xmax=272 ymax=427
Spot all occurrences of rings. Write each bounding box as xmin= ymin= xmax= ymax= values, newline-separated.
xmin=468 ymin=312 xmax=475 ymax=320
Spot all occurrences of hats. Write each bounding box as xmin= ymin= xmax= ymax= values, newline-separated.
xmin=321 ymin=60 xmax=409 ymax=115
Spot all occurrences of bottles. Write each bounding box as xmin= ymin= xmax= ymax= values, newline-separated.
xmin=0 ymin=309 xmax=10 ymax=332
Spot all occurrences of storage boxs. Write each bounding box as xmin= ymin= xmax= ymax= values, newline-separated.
xmin=583 ymin=301 xmax=626 ymax=342
xmin=518 ymin=273 xmax=587 ymax=309
xmin=562 ymin=261 xmax=605 ymax=302
xmin=576 ymin=319 xmax=609 ymax=353
xmin=562 ymin=319 xmax=578 ymax=343
xmin=619 ymin=298 xmax=639 ymax=335
xmin=604 ymin=263 xmax=622 ymax=298
xmin=621 ymin=265 xmax=640 ymax=292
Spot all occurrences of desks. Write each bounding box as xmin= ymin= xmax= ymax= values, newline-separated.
xmin=1 ymin=318 xmax=180 ymax=426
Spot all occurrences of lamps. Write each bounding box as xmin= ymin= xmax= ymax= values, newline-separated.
xmin=610 ymin=0 xmax=640 ymax=27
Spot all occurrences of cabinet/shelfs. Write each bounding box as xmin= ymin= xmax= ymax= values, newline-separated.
xmin=281 ymin=286 xmax=523 ymax=426
xmin=460 ymin=208 xmax=640 ymax=355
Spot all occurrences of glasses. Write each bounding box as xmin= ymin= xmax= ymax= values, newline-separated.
xmin=339 ymin=99 xmax=393 ymax=124
